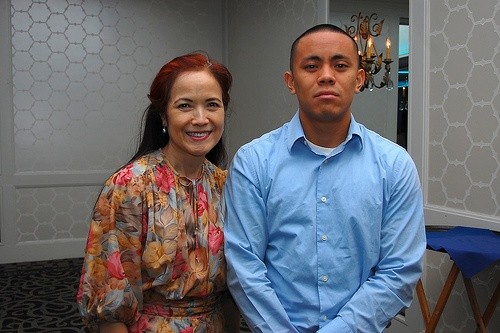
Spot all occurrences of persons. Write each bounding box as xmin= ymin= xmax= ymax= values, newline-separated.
xmin=77 ymin=51 xmax=233 ymax=333
xmin=223 ymin=22 xmax=427 ymax=333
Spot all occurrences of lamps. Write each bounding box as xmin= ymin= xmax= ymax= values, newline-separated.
xmin=343 ymin=12 xmax=394 ymax=92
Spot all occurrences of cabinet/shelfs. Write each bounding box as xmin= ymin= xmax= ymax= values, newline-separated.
xmin=396 ymin=54 xmax=409 ymax=135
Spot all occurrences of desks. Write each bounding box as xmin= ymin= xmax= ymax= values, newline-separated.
xmin=416 ymin=225 xmax=500 ymax=333
